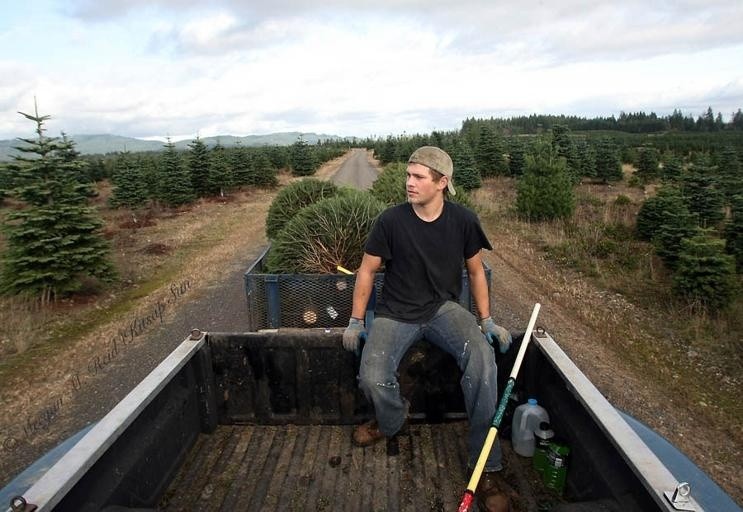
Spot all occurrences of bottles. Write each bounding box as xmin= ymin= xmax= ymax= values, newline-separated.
xmin=544 ymin=437 xmax=569 ymax=489
xmin=531 ymin=421 xmax=554 ymax=476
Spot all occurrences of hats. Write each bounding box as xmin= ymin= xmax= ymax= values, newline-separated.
xmin=407 ymin=145 xmax=457 ymax=197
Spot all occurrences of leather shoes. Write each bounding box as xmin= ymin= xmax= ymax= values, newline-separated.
xmin=466 ymin=468 xmax=510 ymax=512
xmin=352 ymin=417 xmax=385 ymax=447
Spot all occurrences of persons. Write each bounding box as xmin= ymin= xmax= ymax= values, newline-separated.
xmin=340 ymin=144 xmax=514 ymax=511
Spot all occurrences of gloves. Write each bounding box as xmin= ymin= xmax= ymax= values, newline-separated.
xmin=342 ymin=317 xmax=368 ymax=357
xmin=479 ymin=315 xmax=513 ymax=354
